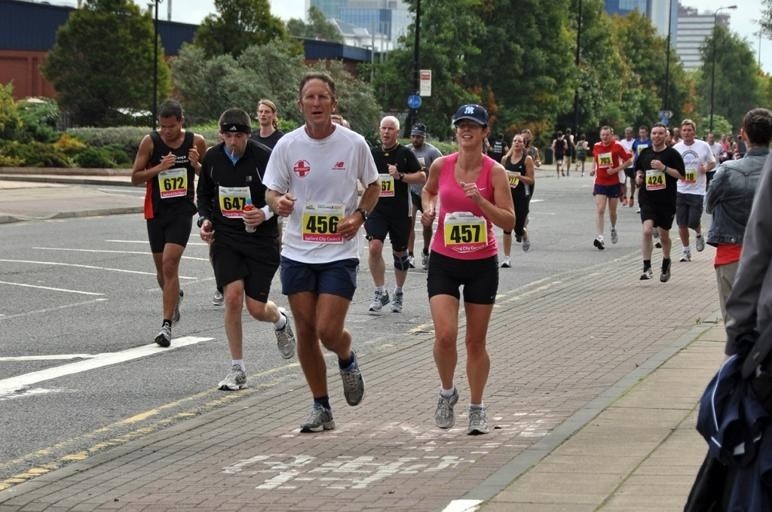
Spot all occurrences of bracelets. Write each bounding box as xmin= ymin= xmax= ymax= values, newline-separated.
xmin=196 ymin=215 xmax=207 ymax=226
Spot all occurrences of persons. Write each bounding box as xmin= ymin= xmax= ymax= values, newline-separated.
xmin=262 ymin=72 xmax=381 ymax=433
xmin=128 ymin=99 xmax=208 ymax=347
xmin=402 ymin=122 xmax=442 ymax=270
xmin=487 ymin=125 xmax=590 ymax=267
xmin=365 ymin=116 xmax=426 ymax=314
xmin=586 ymin=125 xmax=746 ymax=283
xmin=705 ymin=109 xmax=771 ymax=335
xmin=724 ymin=1 xmax=771 ymax=362
xmin=420 ymin=103 xmax=516 ymax=434
xmin=211 ymin=98 xmax=351 ymax=305
xmin=196 ymin=109 xmax=295 ymax=391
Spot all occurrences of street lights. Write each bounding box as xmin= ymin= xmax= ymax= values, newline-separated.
xmin=710 ymin=4 xmax=738 ymax=141
xmin=151 ymin=0 xmax=163 ymax=133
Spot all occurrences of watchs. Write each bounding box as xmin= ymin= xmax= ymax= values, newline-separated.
xmin=353 ymin=208 xmax=371 ymax=220
xmin=399 ymin=171 xmax=404 ymax=181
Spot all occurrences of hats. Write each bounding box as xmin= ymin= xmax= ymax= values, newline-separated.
xmin=452 ymin=104 xmax=489 ymax=125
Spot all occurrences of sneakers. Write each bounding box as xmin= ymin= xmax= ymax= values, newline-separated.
xmin=593 ymin=235 xmax=606 ymax=250
xmin=272 ymin=306 xmax=297 ymax=360
xmin=298 ymin=401 xmax=336 ymax=434
xmin=609 ymin=226 xmax=619 ymax=244
xmin=169 ymin=289 xmax=184 ymax=324
xmin=636 ymin=204 xmax=641 ymax=214
xmin=521 ymin=236 xmax=531 ymax=253
xmin=153 ymin=322 xmax=172 ymax=347
xmin=679 ymin=250 xmax=692 ymax=262
xmin=622 ymin=195 xmax=629 ymax=206
xmin=217 ymin=364 xmax=248 ymax=391
xmin=654 ymin=238 xmax=662 ymax=248
xmin=337 ymin=350 xmax=365 ymax=407
xmin=652 ymin=227 xmax=658 ymax=238
xmin=406 ymin=255 xmax=416 ymax=269
xmin=390 ymin=290 xmax=405 ymax=314
xmin=659 ymin=260 xmax=672 ymax=283
xmin=420 ymin=249 xmax=430 ymax=271
xmin=211 ymin=288 xmax=225 ymax=306
xmin=695 ymin=233 xmax=705 ymax=252
xmin=640 ymin=266 xmax=654 ymax=280
xmin=628 ymin=196 xmax=635 ymax=208
xmin=367 ymin=288 xmax=391 ymax=313
xmin=433 ymin=386 xmax=460 ymax=429
xmin=466 ymin=406 xmax=490 ymax=435
xmin=500 ymin=255 xmax=513 ymax=269
